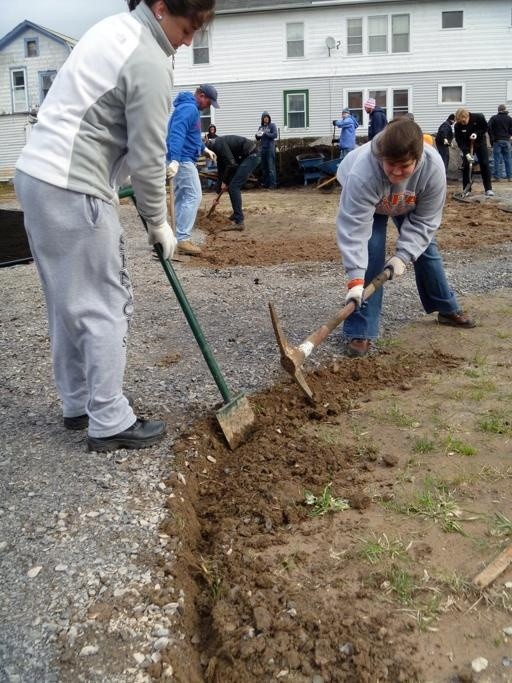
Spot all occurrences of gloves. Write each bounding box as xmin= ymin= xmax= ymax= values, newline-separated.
xmin=165 ymin=160 xmax=179 ymax=179
xmin=466 ymin=153 xmax=476 ymax=162
xmin=470 ymin=133 xmax=477 ymax=140
xmin=444 ymin=138 xmax=449 ymax=145
xmin=332 ymin=119 xmax=338 ymax=126
xmin=383 ymin=255 xmax=406 ymax=280
xmin=145 ymin=219 xmax=178 ymax=260
xmin=204 ymin=147 xmax=217 ymax=162
xmin=332 ymin=138 xmax=337 ymax=143
xmin=345 ymin=284 xmax=368 ymax=314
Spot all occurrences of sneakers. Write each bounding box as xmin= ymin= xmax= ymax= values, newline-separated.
xmin=222 ymin=223 xmax=244 ymax=231
xmin=177 ymin=240 xmax=203 ymax=254
xmin=492 ymin=179 xmax=500 ymax=182
xmin=485 ymin=190 xmax=496 ymax=196
xmin=508 ymin=178 xmax=512 ymax=182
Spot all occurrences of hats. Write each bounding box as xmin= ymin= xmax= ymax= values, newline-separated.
xmin=342 ymin=107 xmax=351 ymax=114
xmin=364 ymin=98 xmax=376 ymax=109
xmin=199 ymin=83 xmax=221 ymax=109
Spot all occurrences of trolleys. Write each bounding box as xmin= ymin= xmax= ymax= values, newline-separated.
xmin=294 ymin=150 xmax=346 ymax=193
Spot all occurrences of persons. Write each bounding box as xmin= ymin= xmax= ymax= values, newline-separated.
xmin=333 ymin=107 xmax=360 ymax=159
xmin=435 ymin=112 xmax=455 ymax=177
xmin=454 ymin=108 xmax=495 ymax=197
xmin=255 ymin=110 xmax=278 ymax=189
xmin=12 ymin=1 xmax=217 ymax=452
xmin=204 ymin=135 xmax=260 ymax=231
xmin=335 ymin=119 xmax=479 ymax=357
xmin=365 ymin=97 xmax=388 ymax=141
xmin=166 ymin=84 xmax=221 ymax=253
xmin=206 ymin=124 xmax=218 ymax=191
xmin=488 ymin=104 xmax=512 ymax=182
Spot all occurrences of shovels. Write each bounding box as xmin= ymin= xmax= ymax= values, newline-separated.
xmin=117 ymin=185 xmax=262 ymax=452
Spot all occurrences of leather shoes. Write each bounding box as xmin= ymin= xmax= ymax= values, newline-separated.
xmin=62 ymin=412 xmax=166 ymax=452
xmin=437 ymin=310 xmax=477 ymax=328
xmin=347 ymin=337 xmax=370 ymax=358
